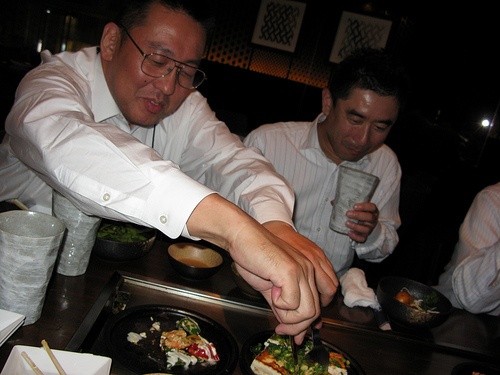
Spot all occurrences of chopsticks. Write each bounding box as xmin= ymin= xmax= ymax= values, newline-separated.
xmin=22 ymin=339 xmax=66 ymax=375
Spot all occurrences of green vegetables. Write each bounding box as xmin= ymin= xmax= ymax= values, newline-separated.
xmin=250 ymin=333 xmax=350 ymax=375
xmin=417 ymin=292 xmax=442 ymax=311
xmin=97 ymin=223 xmax=148 ymax=242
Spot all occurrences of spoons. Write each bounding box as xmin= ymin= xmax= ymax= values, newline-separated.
xmin=304 ymin=325 xmax=330 ymax=366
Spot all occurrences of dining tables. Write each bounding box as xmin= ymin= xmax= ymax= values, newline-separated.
xmin=0 ymin=241 xmax=500 ymax=375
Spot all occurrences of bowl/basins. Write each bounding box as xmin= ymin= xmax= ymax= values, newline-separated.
xmin=167 ymin=242 xmax=225 ymax=280
xmin=231 ymin=262 xmax=264 ymax=298
xmin=451 ymin=362 xmax=500 ymax=375
xmin=95 ymin=218 xmax=158 ymax=261
xmin=378 ymin=279 xmax=452 ymax=330
xmin=239 ymin=330 xmax=365 ymax=375
xmin=103 ymin=304 xmax=238 ymax=375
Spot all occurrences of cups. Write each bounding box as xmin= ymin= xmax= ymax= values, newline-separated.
xmin=52 ymin=188 xmax=102 ymax=276
xmin=0 ymin=210 xmax=66 ymax=326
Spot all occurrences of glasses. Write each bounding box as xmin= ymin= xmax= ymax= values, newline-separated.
xmin=120 ymin=26 xmax=207 ymax=91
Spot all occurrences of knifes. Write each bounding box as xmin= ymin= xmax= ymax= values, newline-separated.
xmin=290 ymin=334 xmax=298 ymax=366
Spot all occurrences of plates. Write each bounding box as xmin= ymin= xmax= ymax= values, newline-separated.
xmin=0 ymin=309 xmax=25 ymax=347
xmin=1 ymin=345 xmax=112 ymax=375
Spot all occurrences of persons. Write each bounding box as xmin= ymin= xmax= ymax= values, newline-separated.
xmin=453 ymin=183 xmax=500 ymax=315
xmin=0 ymin=0 xmax=400 ymax=344
